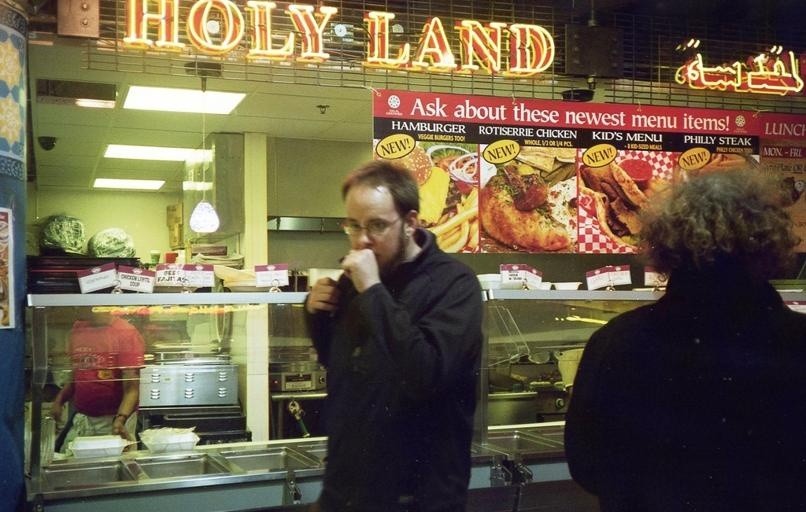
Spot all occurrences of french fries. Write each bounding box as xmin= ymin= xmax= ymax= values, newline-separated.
xmin=425 ymin=189 xmax=479 ymax=253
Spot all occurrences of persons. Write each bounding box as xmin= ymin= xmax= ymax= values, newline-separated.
xmin=50 ymin=307 xmax=145 ymax=453
xmin=564 ymin=166 xmax=805 ymax=511
xmin=302 ymin=161 xmax=481 ymax=511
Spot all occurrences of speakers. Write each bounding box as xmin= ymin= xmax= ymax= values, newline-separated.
xmin=565 ymin=23 xmax=625 ymax=76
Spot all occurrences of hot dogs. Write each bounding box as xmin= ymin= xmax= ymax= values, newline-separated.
xmin=578 ymin=162 xmax=651 ymax=251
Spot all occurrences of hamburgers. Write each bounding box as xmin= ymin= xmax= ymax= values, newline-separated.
xmin=374 ymin=147 xmax=446 ymax=228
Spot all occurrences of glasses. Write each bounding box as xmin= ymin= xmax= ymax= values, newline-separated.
xmin=342 ymin=218 xmax=402 ymax=238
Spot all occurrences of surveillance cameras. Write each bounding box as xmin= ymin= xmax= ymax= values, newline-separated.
xmin=38 ymin=136 xmax=56 ymax=150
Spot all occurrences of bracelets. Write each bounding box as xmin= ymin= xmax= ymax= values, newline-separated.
xmin=113 ymin=414 xmax=128 ymax=423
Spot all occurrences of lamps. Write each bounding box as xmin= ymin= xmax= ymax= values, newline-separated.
xmin=186 ymin=61 xmax=222 ymax=233
